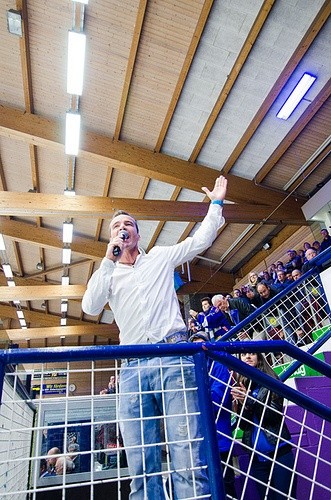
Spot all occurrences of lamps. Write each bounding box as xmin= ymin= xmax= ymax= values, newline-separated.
xmin=62 ymin=221 xmax=74 ymax=243
xmin=64 ymin=111 xmax=82 ymax=157
xmin=276 ymin=72 xmax=316 ymax=121
xmin=282 ymin=135 xmax=331 ymax=191
xmin=67 ymin=28 xmax=86 ymax=96
xmin=263 ymin=243 xmax=272 ymax=251
xmin=220 ymin=224 xmax=254 ymax=261
xmin=7 ymin=8 xmax=23 ymax=37
xmin=1 ymin=262 xmax=70 ymax=342
xmin=62 ymin=247 xmax=71 ymax=265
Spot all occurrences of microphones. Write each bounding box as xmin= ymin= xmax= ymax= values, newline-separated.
xmin=113 ymin=229 xmax=130 ymax=255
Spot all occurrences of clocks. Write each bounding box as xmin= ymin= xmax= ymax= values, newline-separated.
xmin=68 ymin=383 xmax=78 ymax=392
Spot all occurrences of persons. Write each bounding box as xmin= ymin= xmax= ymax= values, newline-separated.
xmin=81 ymin=175 xmax=227 ymax=500
xmin=231 ymin=352 xmax=297 ymax=500
xmin=186 ymin=293 xmax=273 ymax=368
xmin=96 ymin=423 xmax=128 ymax=470
xmin=233 ymin=229 xmax=331 ymax=345
xmin=40 ymin=434 xmax=82 ymax=477
xmin=100 ymin=374 xmax=120 ymax=395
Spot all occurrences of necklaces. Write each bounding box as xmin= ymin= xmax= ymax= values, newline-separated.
xmin=119 ymin=260 xmax=136 ymax=266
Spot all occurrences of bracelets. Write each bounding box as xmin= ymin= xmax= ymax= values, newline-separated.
xmin=211 ymin=199 xmax=224 ymax=207
